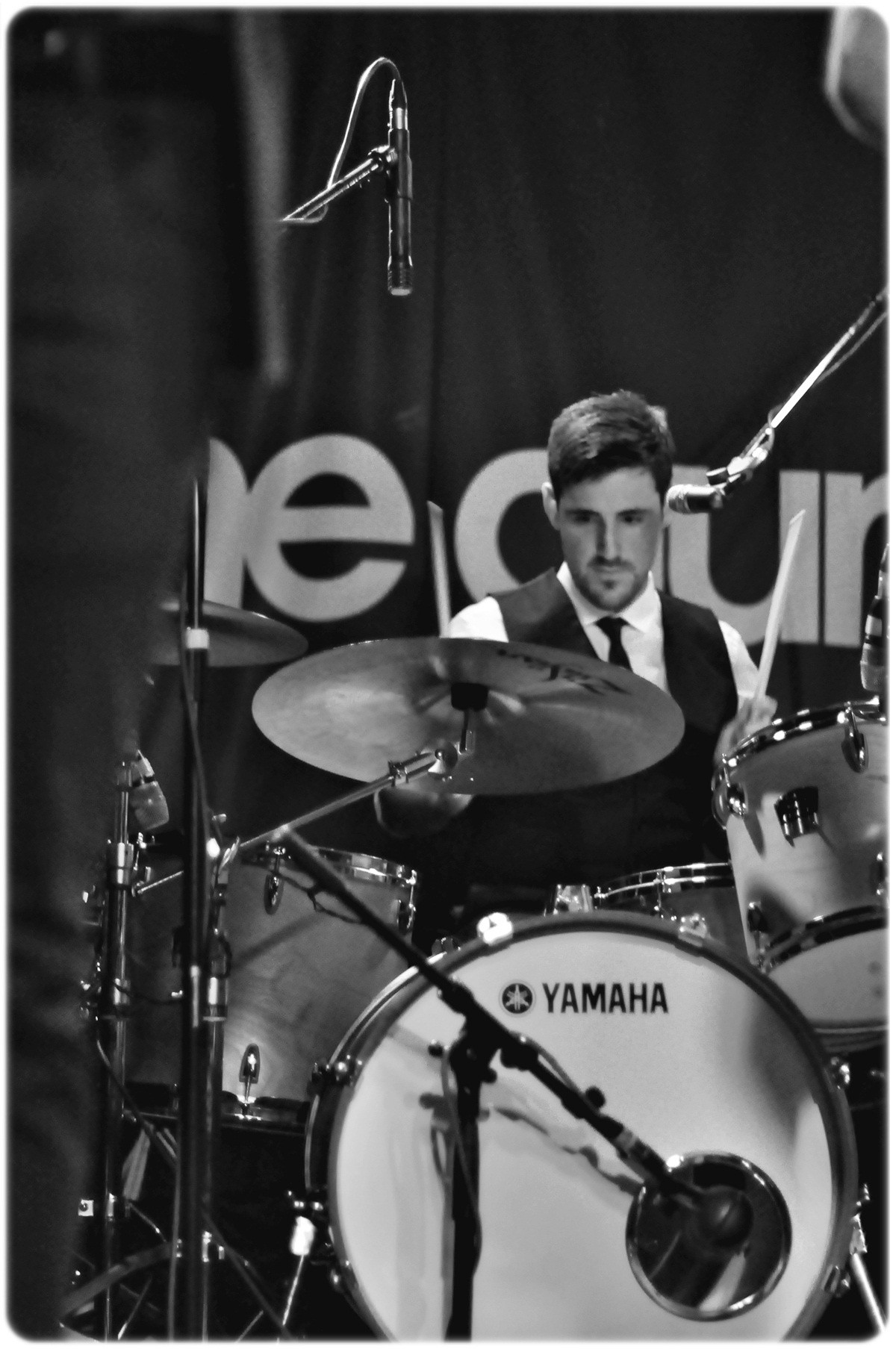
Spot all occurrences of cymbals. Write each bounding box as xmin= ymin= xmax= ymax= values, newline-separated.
xmin=143 ymin=580 xmax=308 ymax=668
xmin=252 ymin=635 xmax=686 ymax=795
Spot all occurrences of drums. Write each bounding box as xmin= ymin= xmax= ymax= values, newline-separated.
xmin=584 ymin=862 xmax=751 ymax=963
xmin=117 ymin=834 xmax=417 ymax=1137
xmin=711 ymin=702 xmax=890 ymax=1046
xmin=296 ymin=909 xmax=861 ymax=1343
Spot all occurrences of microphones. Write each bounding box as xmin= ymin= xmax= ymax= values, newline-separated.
xmin=667 ymin=485 xmax=723 ymax=515
xmin=668 ymin=1177 xmax=755 ymax=1248
xmin=129 ymin=751 xmax=169 ymax=829
xmin=385 ymin=77 xmax=413 ymax=295
xmin=860 ymin=545 xmax=890 ymax=693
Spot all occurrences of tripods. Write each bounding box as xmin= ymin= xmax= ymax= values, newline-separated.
xmin=54 ymin=703 xmax=477 ymax=1342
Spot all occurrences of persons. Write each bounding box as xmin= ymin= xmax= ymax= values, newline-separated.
xmin=377 ymin=390 xmax=778 ymax=915
